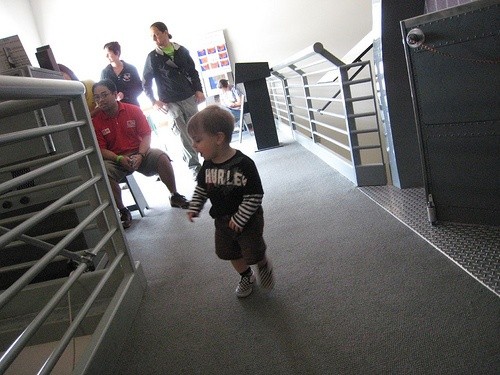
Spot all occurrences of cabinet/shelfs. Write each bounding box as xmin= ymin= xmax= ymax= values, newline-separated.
xmin=0 ymin=67 xmax=109 ymax=291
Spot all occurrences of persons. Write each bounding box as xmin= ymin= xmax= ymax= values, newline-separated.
xmin=142 ymin=22 xmax=205 ymax=181
xmin=218 ymin=79 xmax=241 ymax=123
xmin=92 ymin=79 xmax=191 ymax=229
xmin=187 ymin=105 xmax=273 ymax=297
xmin=100 ymin=42 xmax=144 ymax=107
xmin=58 ymin=64 xmax=96 ymax=114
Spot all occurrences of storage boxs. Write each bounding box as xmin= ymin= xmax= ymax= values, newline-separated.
xmin=400 ymin=1 xmax=500 ymax=226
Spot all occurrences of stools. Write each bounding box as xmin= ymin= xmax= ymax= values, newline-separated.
xmin=121 ymin=173 xmax=149 ymax=217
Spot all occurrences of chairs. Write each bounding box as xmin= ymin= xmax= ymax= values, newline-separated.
xmin=229 ymin=96 xmax=251 ymax=143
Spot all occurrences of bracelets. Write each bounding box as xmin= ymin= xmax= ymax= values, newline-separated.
xmin=136 ymin=152 xmax=146 ymax=165
xmin=114 ymin=154 xmax=123 ymax=163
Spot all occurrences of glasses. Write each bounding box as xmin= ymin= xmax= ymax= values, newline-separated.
xmin=92 ymin=90 xmax=117 ymax=101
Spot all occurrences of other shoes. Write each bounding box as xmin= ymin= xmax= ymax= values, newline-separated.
xmin=122 ymin=183 xmax=129 ymax=189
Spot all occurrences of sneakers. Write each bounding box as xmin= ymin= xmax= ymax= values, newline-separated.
xmin=193 ymin=170 xmax=200 ymax=180
xmin=234 ymin=269 xmax=256 ymax=297
xmin=119 ymin=209 xmax=132 ymax=229
xmin=257 ymin=259 xmax=275 ymax=289
xmin=169 ymin=194 xmax=190 ymax=209
